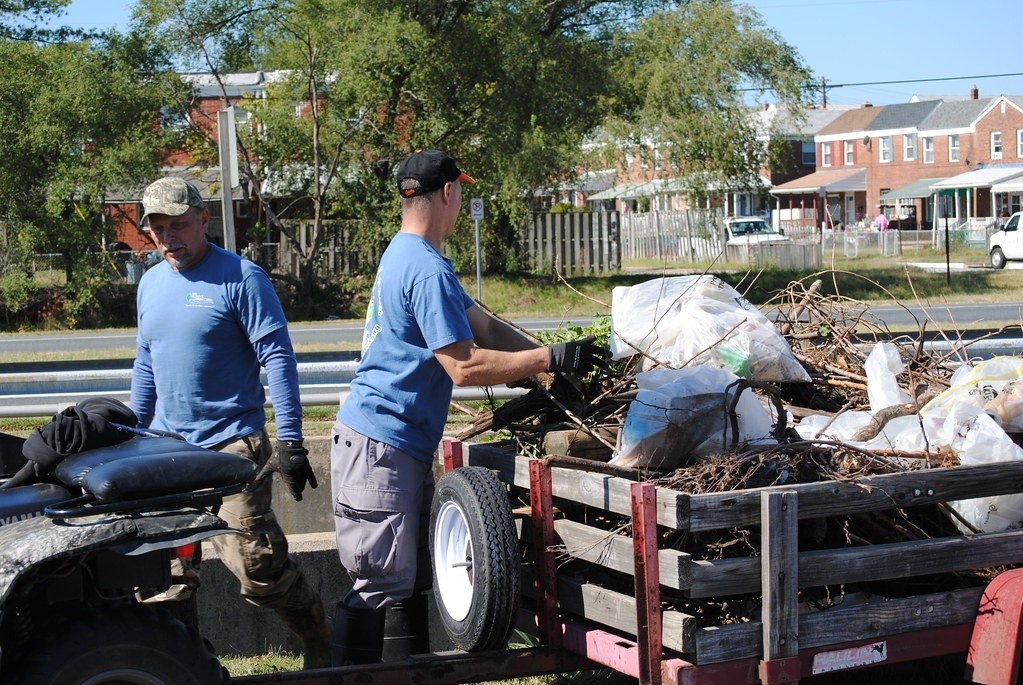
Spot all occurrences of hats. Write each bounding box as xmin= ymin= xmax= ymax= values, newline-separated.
xmin=396 ymin=148 xmax=476 ymax=198
xmin=140 ymin=177 xmax=204 ymax=225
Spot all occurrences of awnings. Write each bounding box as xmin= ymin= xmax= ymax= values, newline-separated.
xmin=879 ymin=166 xmax=1023 ymax=199
xmin=548 ymin=169 xmax=614 ymax=190
xmin=587 ymin=168 xmax=771 ymax=200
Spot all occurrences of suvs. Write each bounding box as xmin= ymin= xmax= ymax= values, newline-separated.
xmin=988 ymin=209 xmax=1023 ymax=269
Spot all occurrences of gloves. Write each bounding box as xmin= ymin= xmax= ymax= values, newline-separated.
xmin=544 ymin=335 xmax=613 ymax=380
xmin=549 ymin=371 xmax=588 ymax=401
xmin=249 ymin=440 xmax=318 ymax=502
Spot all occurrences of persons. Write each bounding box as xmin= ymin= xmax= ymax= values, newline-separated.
xmin=334 ymin=147 xmax=613 ymax=681
xmin=120 ymin=176 xmax=333 ymax=669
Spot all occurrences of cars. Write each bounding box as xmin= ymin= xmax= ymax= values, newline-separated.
xmin=720 ymin=215 xmax=793 ymax=247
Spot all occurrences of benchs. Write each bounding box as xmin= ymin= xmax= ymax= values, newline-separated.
xmin=42 ymin=435 xmax=256 ymax=522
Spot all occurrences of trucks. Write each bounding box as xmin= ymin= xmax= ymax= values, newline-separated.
xmin=0 ymin=339 xmax=1023 ymax=684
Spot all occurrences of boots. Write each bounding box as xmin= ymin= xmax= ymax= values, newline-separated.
xmin=382 ymin=594 xmax=430 ymax=661
xmin=331 ymin=602 xmax=386 ymax=685
